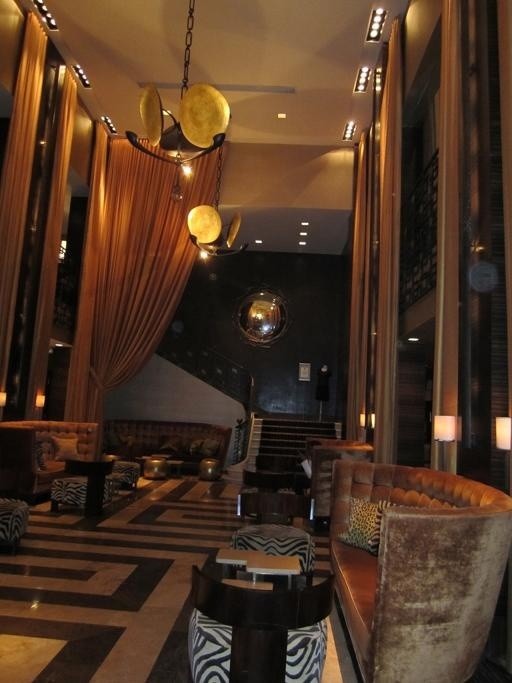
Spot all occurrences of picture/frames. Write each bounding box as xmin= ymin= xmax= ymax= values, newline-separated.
xmin=296 ymin=360 xmax=312 ymax=384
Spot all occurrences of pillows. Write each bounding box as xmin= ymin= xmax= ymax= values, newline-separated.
xmin=158 ymin=435 xmax=182 ymax=453
xmin=188 ymin=440 xmax=205 ymax=457
xmin=367 ymin=499 xmax=428 ymax=557
xmin=51 ymin=436 xmax=81 ymax=461
xmin=336 ymin=496 xmax=380 ymax=550
xmin=198 ymin=438 xmax=220 ymax=458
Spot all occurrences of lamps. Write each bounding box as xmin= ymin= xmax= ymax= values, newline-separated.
xmin=186 ymin=145 xmax=250 ymax=257
xmin=125 ymin=1 xmax=231 ymax=203
xmin=433 ymin=416 xmax=456 ymax=443
xmin=495 ymin=416 xmax=512 ymax=452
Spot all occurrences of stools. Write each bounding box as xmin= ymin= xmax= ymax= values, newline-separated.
xmin=143 ymin=457 xmax=166 ymax=480
xmin=199 ymin=457 xmax=222 ymax=481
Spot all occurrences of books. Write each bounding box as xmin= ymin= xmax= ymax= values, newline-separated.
xmin=244 ymin=550 xmax=301 ymax=576
xmin=216 ymin=547 xmax=266 ymax=564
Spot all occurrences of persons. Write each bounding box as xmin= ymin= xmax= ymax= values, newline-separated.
xmin=295 ymin=439 xmax=323 ymax=495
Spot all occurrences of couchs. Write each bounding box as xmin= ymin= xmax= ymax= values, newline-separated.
xmin=305 ymin=437 xmax=375 ymax=520
xmin=329 ymin=459 xmax=511 ymax=683
xmin=0 ymin=421 xmax=103 ymax=496
xmin=97 ymin=421 xmax=232 ymax=475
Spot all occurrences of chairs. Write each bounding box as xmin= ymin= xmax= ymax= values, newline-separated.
xmin=242 ymin=468 xmax=311 ymax=492
xmin=105 ymin=461 xmax=141 ymax=497
xmin=230 ymin=492 xmax=314 ymax=586
xmin=0 ymin=498 xmax=30 ymax=555
xmin=186 ymin=564 xmax=332 ymax=683
xmin=49 ymin=459 xmax=114 ymax=516
